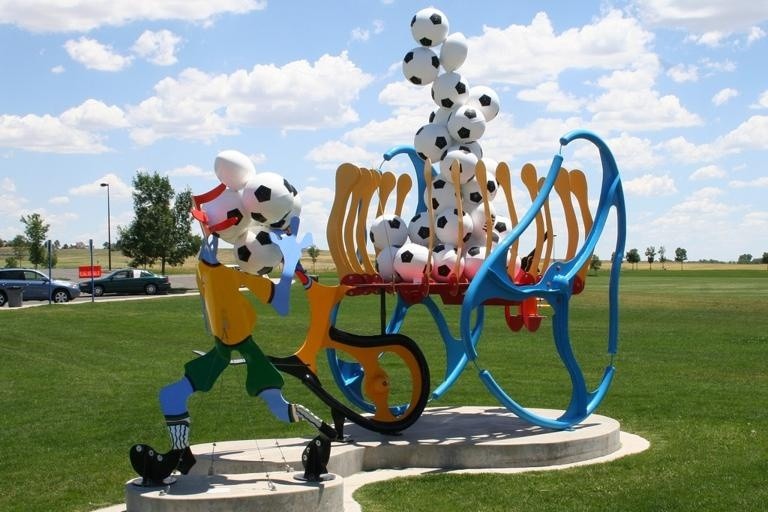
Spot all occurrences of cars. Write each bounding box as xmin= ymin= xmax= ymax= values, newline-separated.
xmin=0 ymin=268 xmax=81 ymax=307
xmin=78 ymin=268 xmax=170 ymax=296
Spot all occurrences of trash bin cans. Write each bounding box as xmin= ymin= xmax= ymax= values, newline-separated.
xmin=5 ymin=286 xmax=23 ymax=307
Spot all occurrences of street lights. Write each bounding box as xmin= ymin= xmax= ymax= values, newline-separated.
xmin=100 ymin=183 xmax=111 ymax=271
